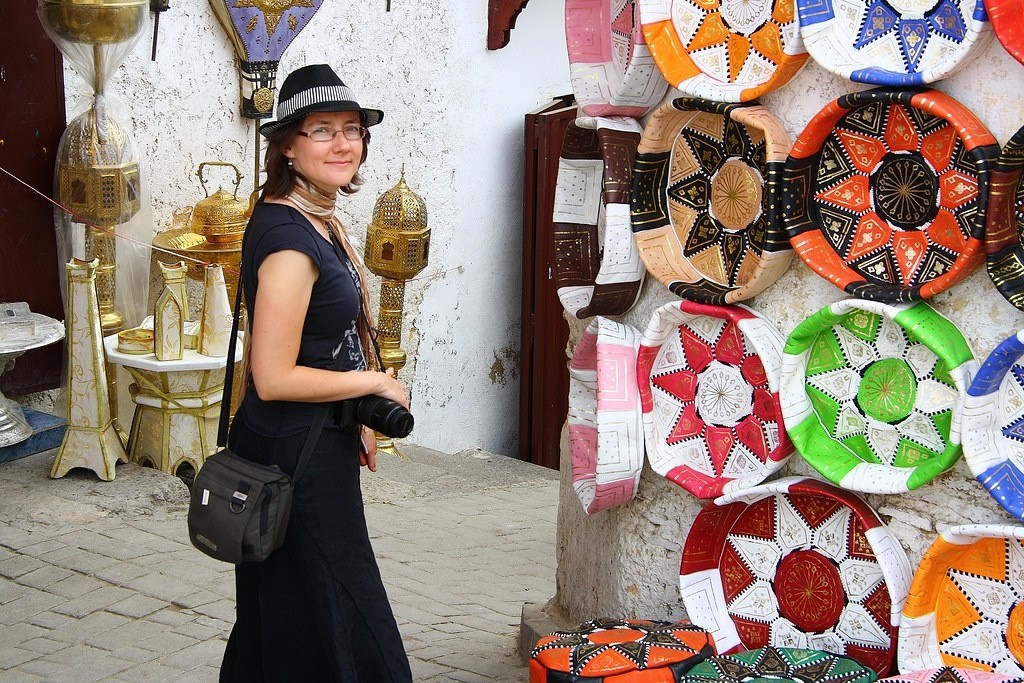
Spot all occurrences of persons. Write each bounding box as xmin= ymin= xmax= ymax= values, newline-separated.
xmin=218 ymin=64 xmax=412 ymax=683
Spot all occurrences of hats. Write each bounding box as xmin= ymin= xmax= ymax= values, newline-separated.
xmin=259 ymin=64 xmax=384 ymax=141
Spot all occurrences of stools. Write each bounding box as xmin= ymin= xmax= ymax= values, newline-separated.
xmin=679 ymin=646 xmax=878 ymax=683
xmin=528 ymin=617 xmax=719 ymax=683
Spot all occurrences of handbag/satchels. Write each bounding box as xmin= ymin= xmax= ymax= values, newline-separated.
xmin=187 ymin=449 xmax=294 ymax=565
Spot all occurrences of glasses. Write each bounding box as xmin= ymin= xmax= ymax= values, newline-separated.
xmin=295 ymin=124 xmax=369 ymax=142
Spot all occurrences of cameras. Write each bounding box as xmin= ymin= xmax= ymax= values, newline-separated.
xmin=333 ymin=394 xmax=415 ymax=439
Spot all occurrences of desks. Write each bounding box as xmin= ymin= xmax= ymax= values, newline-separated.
xmin=103 ymin=324 xmax=248 ymax=480
